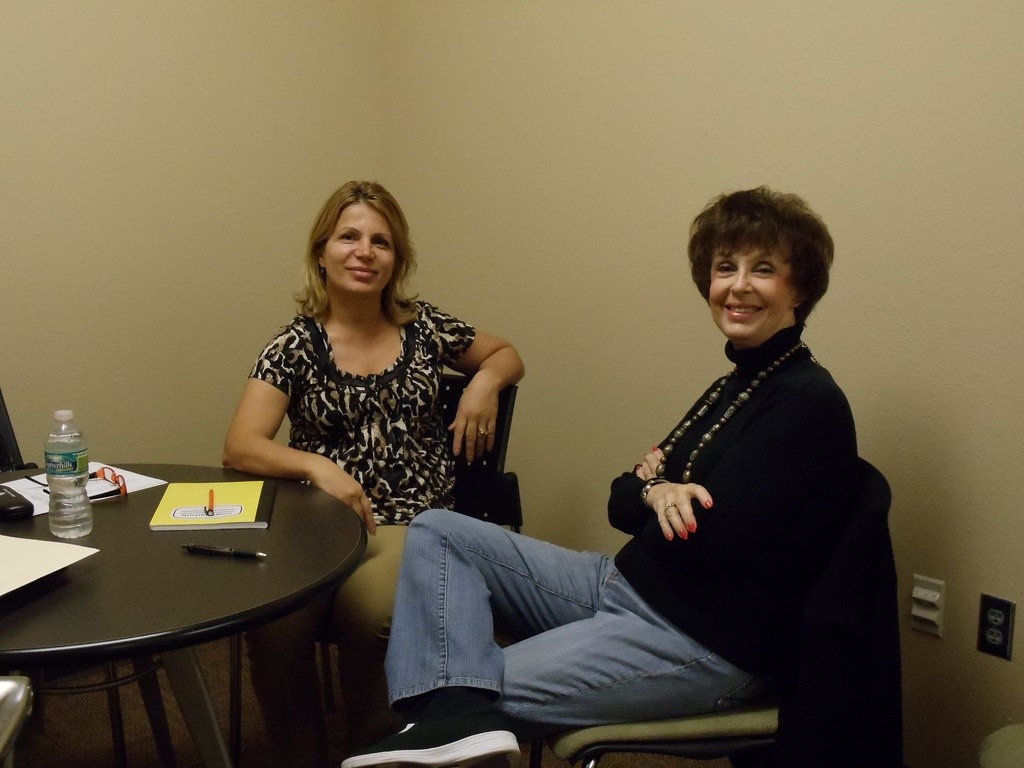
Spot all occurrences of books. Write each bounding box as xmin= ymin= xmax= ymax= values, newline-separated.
xmin=149 ymin=480 xmax=277 ymax=531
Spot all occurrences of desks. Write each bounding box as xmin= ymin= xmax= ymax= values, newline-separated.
xmin=0 ymin=453 xmax=368 ymax=768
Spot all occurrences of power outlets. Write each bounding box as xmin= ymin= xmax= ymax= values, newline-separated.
xmin=972 ymin=592 xmax=1018 ymax=662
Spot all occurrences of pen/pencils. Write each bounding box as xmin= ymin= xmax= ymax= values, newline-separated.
xmin=181 ymin=543 xmax=267 ymax=559
xmin=204 ymin=489 xmax=213 ymax=516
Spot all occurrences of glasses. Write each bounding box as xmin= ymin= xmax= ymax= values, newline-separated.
xmin=23 ymin=467 xmax=127 ymax=499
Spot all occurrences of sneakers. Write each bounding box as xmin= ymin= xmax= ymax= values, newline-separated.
xmin=341 ymin=710 xmax=522 ymax=768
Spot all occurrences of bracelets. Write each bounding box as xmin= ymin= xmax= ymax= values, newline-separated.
xmin=641 ymin=478 xmax=670 ymax=501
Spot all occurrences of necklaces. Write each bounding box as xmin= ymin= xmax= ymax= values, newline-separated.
xmin=655 ymin=340 xmax=804 ymax=483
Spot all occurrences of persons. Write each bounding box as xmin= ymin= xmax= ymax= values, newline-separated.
xmin=221 ymin=180 xmax=524 ymax=768
xmin=338 ymin=179 xmax=858 ymax=768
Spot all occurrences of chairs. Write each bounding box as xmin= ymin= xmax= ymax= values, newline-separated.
xmin=229 ymin=378 xmax=530 ymax=745
xmin=543 ymin=469 xmax=905 ymax=767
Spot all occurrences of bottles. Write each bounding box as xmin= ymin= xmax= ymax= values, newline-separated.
xmin=43 ymin=410 xmax=93 ymax=539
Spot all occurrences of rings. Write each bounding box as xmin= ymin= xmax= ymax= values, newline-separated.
xmin=478 ymin=429 xmax=488 ymax=435
xmin=665 ymin=504 xmax=676 ymax=513
